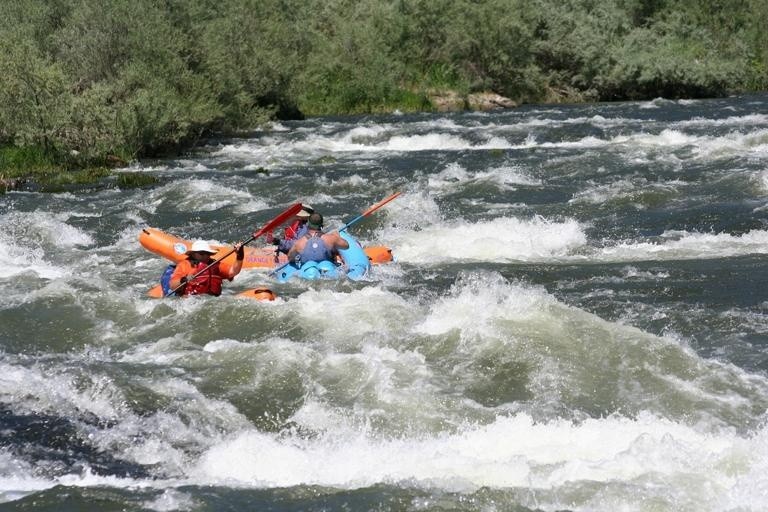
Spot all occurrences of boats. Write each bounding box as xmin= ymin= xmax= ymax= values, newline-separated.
xmin=277 ymin=231 xmax=370 ymax=283
xmin=146 ymin=284 xmax=274 ymax=303
xmin=139 ymin=227 xmax=393 ymax=281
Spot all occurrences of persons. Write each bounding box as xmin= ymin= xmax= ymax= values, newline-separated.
xmin=169 ymin=239 xmax=244 ymax=296
xmin=288 ymin=213 xmax=349 ymax=264
xmin=278 ymin=205 xmax=315 ymax=251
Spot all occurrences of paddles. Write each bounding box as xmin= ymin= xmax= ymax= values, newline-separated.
xmin=268 ymin=190 xmax=401 ymax=276
xmin=164 ymin=202 xmax=303 ymax=299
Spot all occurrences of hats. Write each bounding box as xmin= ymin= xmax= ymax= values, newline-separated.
xmin=296 ymin=204 xmax=314 ymax=217
xmin=185 ymin=240 xmax=218 ymax=255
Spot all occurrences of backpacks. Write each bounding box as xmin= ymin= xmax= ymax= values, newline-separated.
xmin=304 ymin=232 xmax=325 ymax=261
